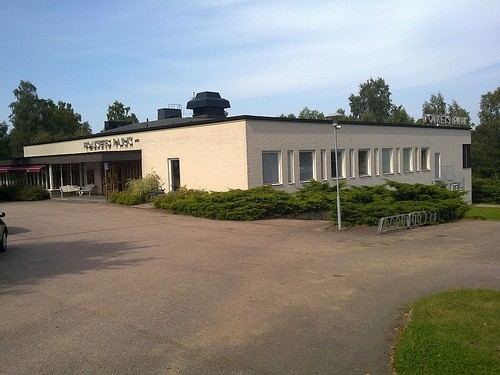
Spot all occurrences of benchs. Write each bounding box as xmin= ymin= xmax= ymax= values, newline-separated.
xmin=58 ymin=184 xmax=81 ymax=197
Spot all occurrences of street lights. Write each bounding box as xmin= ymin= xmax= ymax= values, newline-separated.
xmin=324 ymin=111 xmax=344 ymax=231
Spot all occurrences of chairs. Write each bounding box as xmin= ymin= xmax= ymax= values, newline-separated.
xmin=80 ymin=184 xmax=97 ymax=197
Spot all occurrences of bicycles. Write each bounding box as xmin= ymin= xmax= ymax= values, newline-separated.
xmin=145 ymin=184 xmax=165 ymax=204
